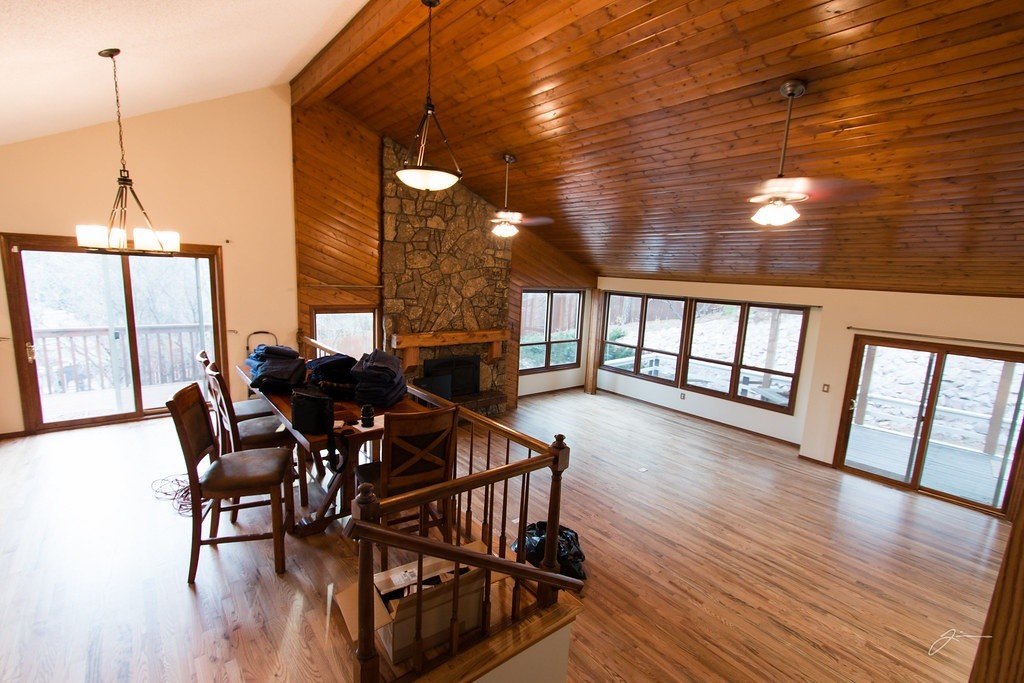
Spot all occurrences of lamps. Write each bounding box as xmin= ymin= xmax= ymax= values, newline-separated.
xmin=747 ymin=79 xmax=809 ymax=226
xmin=489 ymin=153 xmax=523 ymax=238
xmin=396 ymin=0 xmax=463 ymax=191
xmin=75 ymin=49 xmax=180 ymax=257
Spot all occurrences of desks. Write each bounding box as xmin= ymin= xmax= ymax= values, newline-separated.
xmin=236 ymin=365 xmax=430 ymax=538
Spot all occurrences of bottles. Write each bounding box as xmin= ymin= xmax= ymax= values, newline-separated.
xmin=360 ymin=404 xmax=374 ymax=427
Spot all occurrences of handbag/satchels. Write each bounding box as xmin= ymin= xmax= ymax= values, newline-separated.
xmin=304 ymin=353 xmax=361 ymax=392
xmin=291 ymin=390 xmax=348 ymax=472
xmin=509 ymin=521 xmax=588 ymax=581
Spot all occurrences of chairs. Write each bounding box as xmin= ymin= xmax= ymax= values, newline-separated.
xmin=197 ymin=350 xmax=274 ymax=453
xmin=354 ymin=403 xmax=461 ymax=571
xmin=206 ymin=361 xmax=309 ymax=523
xmin=166 ymin=382 xmax=294 ymax=584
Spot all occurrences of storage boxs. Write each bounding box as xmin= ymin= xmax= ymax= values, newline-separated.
xmin=333 ymin=540 xmax=513 ymax=665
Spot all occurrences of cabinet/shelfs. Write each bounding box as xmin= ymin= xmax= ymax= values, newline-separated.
xmin=450 ymin=389 xmax=507 ymax=417
